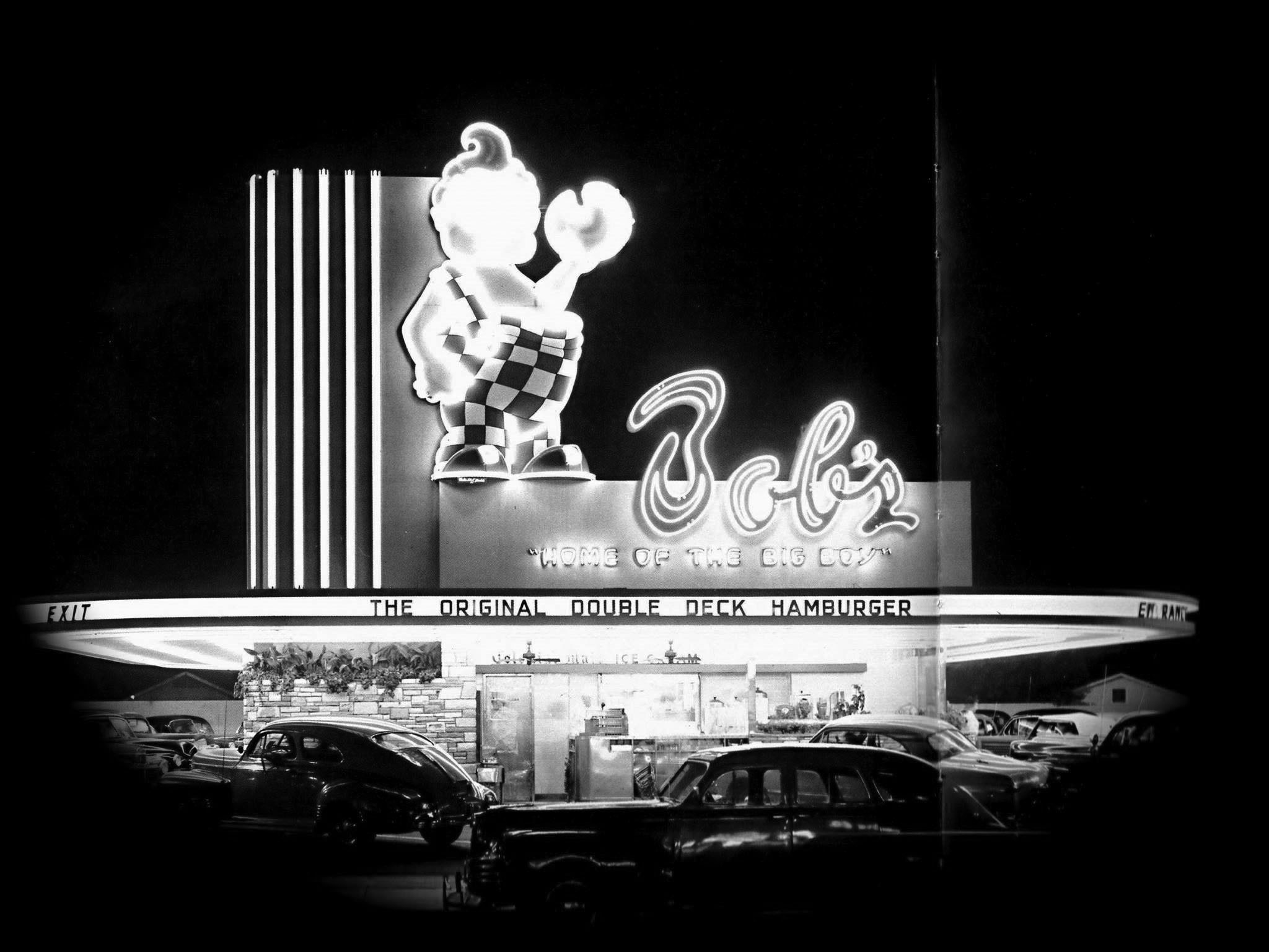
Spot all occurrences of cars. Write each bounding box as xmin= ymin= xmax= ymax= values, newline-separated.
xmin=714 ymin=713 xmax=1055 ymax=828
xmin=466 ymin=741 xmax=1051 ymax=935
xmin=157 ymin=715 xmax=499 ymax=871
xmin=954 ymin=702 xmax=1176 ymax=788
xmin=78 ymin=711 xmax=244 ymax=802
